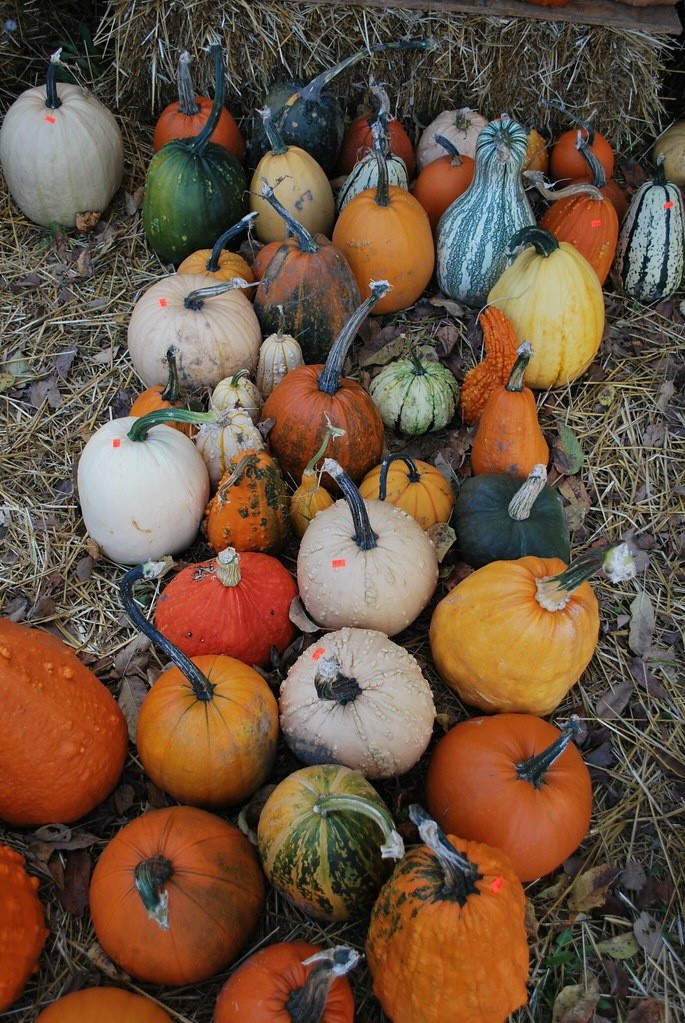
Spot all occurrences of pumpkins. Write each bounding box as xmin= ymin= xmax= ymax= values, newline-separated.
xmin=0 ymin=37 xmax=685 ymax=1023
